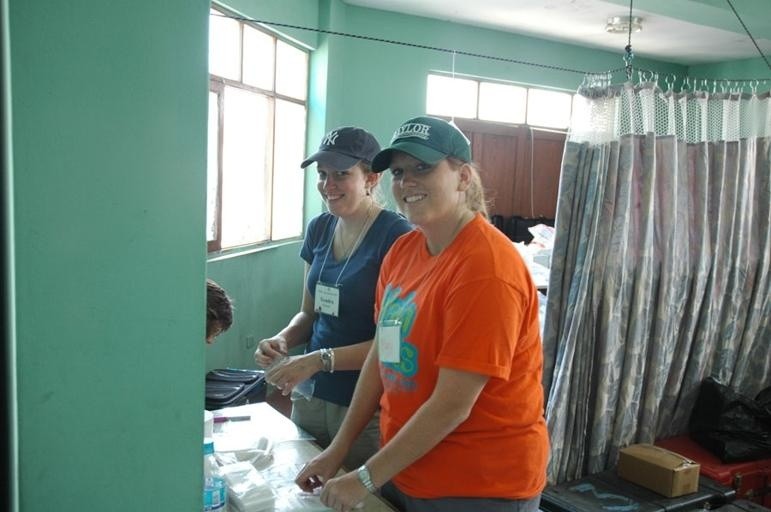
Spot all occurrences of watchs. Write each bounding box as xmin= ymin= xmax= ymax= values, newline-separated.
xmin=357 ymin=465 xmax=378 ymax=496
xmin=319 ymin=348 xmax=331 ymax=373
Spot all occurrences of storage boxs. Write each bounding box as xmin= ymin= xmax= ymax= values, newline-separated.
xmin=618 ymin=443 xmax=701 ymax=497
xmin=206 ymin=368 xmax=266 ymax=411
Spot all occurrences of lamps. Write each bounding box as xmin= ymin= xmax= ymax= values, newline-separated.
xmin=605 ymin=15 xmax=643 ymax=34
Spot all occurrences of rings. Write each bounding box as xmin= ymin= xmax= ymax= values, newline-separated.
xmin=288 ymin=382 xmax=294 ymax=389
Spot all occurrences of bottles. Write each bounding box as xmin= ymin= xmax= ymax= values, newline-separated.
xmin=203 ymin=437 xmax=226 ymax=512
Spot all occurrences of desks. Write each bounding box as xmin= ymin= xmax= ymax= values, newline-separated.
xmin=204 ymin=402 xmax=400 ymax=512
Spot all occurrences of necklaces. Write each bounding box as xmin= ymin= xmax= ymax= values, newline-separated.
xmin=337 ymin=204 xmax=373 ymax=261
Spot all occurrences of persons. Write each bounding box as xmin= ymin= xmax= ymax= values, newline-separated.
xmin=295 ymin=115 xmax=551 ymax=511
xmin=207 ymin=279 xmax=232 ymax=346
xmin=251 ymin=125 xmax=414 ymax=499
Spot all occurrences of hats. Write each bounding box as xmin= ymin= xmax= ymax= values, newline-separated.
xmin=371 ymin=116 xmax=472 ymax=173
xmin=299 ymin=127 xmax=380 ymax=171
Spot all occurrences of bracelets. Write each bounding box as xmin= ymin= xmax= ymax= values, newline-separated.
xmin=328 ymin=349 xmax=335 ymax=373
